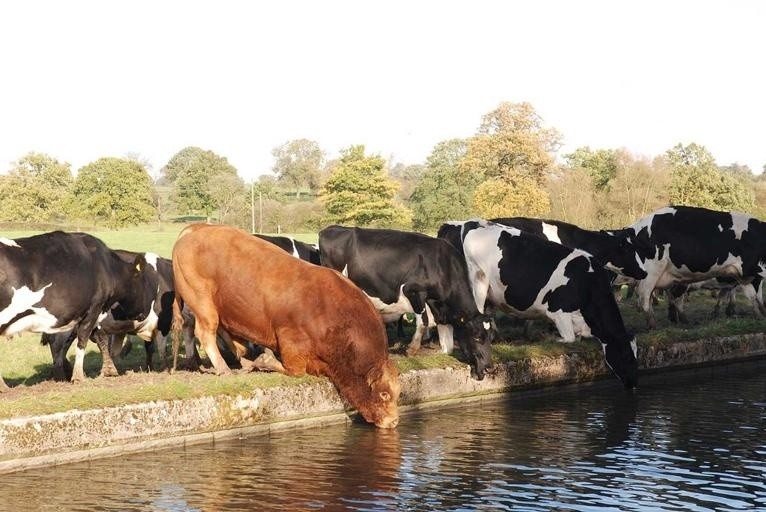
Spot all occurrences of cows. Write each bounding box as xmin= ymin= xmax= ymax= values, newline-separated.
xmin=1 ymin=228 xmax=148 ymax=388
xmin=169 ymin=221 xmax=402 ymax=431
xmin=41 ymin=249 xmax=200 ymax=385
xmin=437 ymin=218 xmax=640 ymax=390
xmin=318 ymin=224 xmax=494 ymax=382
xmin=662 ymin=277 xmax=743 ymax=325
xmin=634 ymin=204 xmax=766 ymax=330
xmin=488 ymin=216 xmax=647 ymax=288
xmin=250 ymin=230 xmax=320 ymax=264
xmin=598 ymin=225 xmax=641 ymax=306
